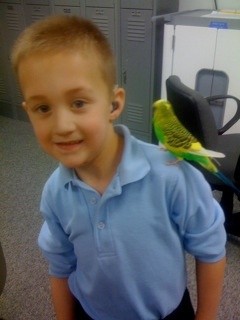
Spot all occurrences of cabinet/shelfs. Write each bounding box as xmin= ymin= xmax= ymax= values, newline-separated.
xmin=2 ymin=1 xmax=179 ymax=146
xmin=158 ymin=8 xmax=238 ymax=137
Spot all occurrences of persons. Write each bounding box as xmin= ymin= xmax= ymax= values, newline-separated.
xmin=10 ymin=15 xmax=228 ymax=320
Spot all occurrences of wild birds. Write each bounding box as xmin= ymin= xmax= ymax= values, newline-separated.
xmin=151 ymin=99 xmax=240 ymax=196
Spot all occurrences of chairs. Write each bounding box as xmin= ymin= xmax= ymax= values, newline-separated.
xmin=165 ymin=75 xmax=240 ymax=246
